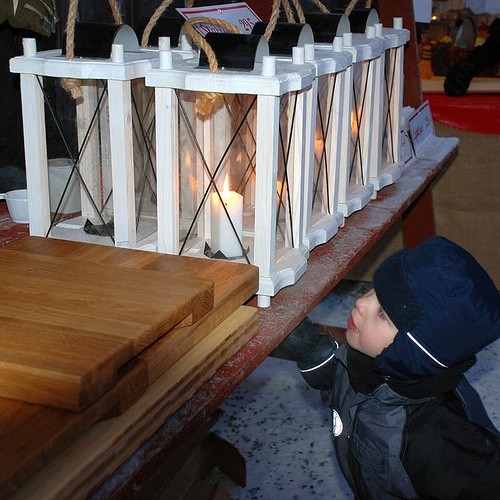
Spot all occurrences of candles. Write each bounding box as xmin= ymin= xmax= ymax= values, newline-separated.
xmin=210 ymin=173 xmax=243 ymax=257
xmin=0 ymin=136 xmax=461 ymax=500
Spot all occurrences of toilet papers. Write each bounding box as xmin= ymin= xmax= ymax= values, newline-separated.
xmin=47 ymin=158 xmax=82 ymax=214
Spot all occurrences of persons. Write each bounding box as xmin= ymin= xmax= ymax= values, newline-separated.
xmin=280 ymin=236 xmax=500 ymax=500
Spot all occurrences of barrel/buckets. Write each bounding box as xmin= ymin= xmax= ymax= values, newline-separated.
xmin=48 ymin=158 xmax=80 ymax=213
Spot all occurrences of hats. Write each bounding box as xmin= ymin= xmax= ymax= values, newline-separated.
xmin=362 ymin=236 xmax=500 ymax=376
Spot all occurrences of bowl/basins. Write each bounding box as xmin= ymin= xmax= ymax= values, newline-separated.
xmin=0 ymin=190 xmax=28 ymax=224
